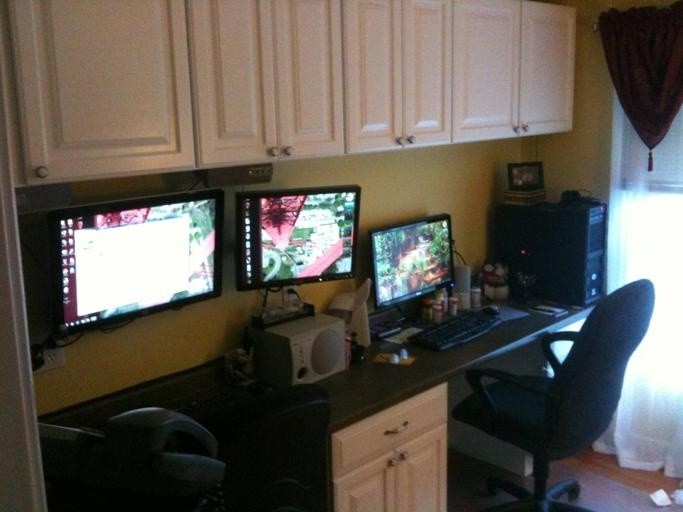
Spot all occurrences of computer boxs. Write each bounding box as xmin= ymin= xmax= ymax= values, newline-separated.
xmin=493 ymin=201 xmax=608 ymax=310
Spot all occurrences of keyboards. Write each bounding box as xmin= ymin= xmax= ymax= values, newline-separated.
xmin=407 ymin=311 xmax=502 ymax=352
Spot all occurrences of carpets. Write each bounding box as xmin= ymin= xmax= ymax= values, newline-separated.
xmin=448 ymin=449 xmax=681 ymax=512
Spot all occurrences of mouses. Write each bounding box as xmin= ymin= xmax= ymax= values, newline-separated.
xmin=480 ymin=304 xmax=500 ymax=316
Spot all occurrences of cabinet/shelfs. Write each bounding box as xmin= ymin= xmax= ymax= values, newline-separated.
xmin=184 ymin=1 xmax=344 ymax=170
xmin=340 ymin=0 xmax=452 ymax=157
xmin=452 ymin=0 xmax=574 ymax=148
xmin=1 ymin=1 xmax=197 ymax=190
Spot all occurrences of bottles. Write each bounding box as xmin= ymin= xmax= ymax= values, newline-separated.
xmin=420 ymin=288 xmax=458 ymax=323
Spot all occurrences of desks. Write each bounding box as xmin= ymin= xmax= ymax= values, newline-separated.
xmin=227 ymin=292 xmax=609 ymax=512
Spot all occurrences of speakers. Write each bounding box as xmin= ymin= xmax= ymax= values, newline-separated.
xmin=247 ymin=311 xmax=346 ymax=388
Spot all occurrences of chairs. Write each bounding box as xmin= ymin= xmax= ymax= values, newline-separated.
xmin=452 ymin=279 xmax=655 ymax=512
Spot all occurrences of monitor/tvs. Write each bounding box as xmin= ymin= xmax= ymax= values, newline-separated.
xmin=46 ymin=188 xmax=226 ymax=339
xmin=368 ymin=213 xmax=455 ymax=322
xmin=235 ymin=184 xmax=361 ymax=292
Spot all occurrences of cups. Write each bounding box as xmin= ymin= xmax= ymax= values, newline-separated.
xmin=454 ymin=289 xmax=470 ymax=311
xmin=471 ymin=288 xmax=483 ymax=308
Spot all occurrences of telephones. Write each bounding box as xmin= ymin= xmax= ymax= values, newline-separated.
xmin=36 ymin=407 xmax=226 ymax=512
